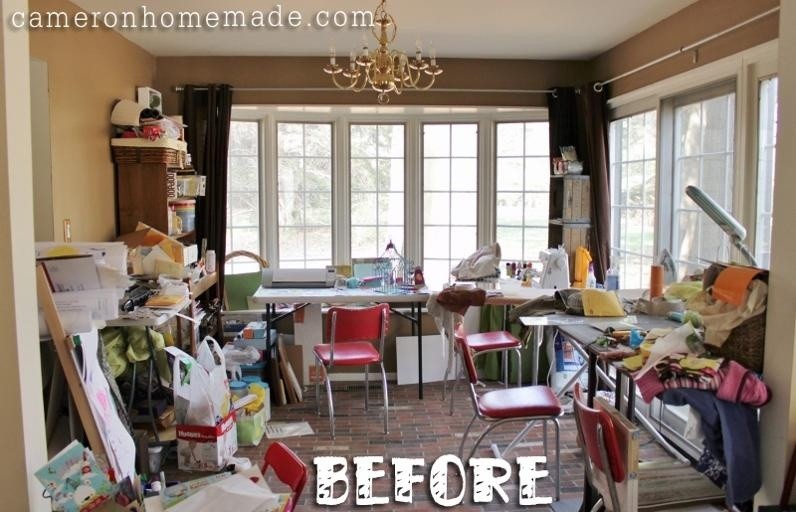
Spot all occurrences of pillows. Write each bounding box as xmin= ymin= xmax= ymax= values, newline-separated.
xmin=582 ymin=288 xmax=627 ymax=316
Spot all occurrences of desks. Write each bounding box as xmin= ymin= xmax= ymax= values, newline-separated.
xmin=491 ymin=315 xmax=726 ymax=510
xmin=253 ymin=284 xmax=430 ymax=400
xmin=483 ymin=295 xmax=539 ymax=385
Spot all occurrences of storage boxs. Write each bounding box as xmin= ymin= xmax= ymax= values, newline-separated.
xmin=703 ymin=262 xmax=769 ymax=377
xmin=237 ymin=406 xmax=267 ymax=447
xmin=177 ymin=410 xmax=240 ymax=473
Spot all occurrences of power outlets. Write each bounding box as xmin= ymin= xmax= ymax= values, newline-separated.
xmin=64 ymin=219 xmax=72 ymax=242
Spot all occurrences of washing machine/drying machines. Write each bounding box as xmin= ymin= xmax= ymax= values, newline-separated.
xmin=651 ymin=266 xmax=663 ymax=299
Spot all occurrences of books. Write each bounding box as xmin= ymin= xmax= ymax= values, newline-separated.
xmin=34 ymin=439 xmax=118 ymax=511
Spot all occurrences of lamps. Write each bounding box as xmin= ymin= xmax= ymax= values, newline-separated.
xmin=321 ymin=0 xmax=443 ymax=104
xmin=686 ymin=186 xmax=758 ymax=268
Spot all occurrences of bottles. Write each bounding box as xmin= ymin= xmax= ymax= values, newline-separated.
xmin=584 ymin=262 xmax=598 ymax=289
xmin=241 ymin=377 xmax=261 ymax=395
xmin=205 ymin=249 xmax=216 ymax=274
xmin=229 ymin=380 xmax=250 ymax=399
xmin=247 ymin=380 xmax=273 ymax=423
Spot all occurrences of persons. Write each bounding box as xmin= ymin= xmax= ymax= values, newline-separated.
xmin=180 ymin=440 xmax=199 ymax=467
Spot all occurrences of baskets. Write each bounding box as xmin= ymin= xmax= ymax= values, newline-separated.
xmin=704 ymin=312 xmax=766 ymax=374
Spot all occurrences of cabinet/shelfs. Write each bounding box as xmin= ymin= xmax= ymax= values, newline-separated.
xmin=548 ymin=174 xmax=591 ymax=284
xmin=111 ymin=137 xmax=197 ymax=246
xmin=107 ymin=263 xmax=223 ymax=445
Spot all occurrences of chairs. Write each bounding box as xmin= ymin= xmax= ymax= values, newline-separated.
xmin=442 ymin=320 xmax=522 ymax=416
xmin=313 ymin=303 xmax=389 ymax=439
xmin=453 ymin=333 xmax=564 ymax=501
xmin=572 ymin=383 xmax=724 ymax=512
xmin=261 ymin=441 xmax=307 ymax=512
